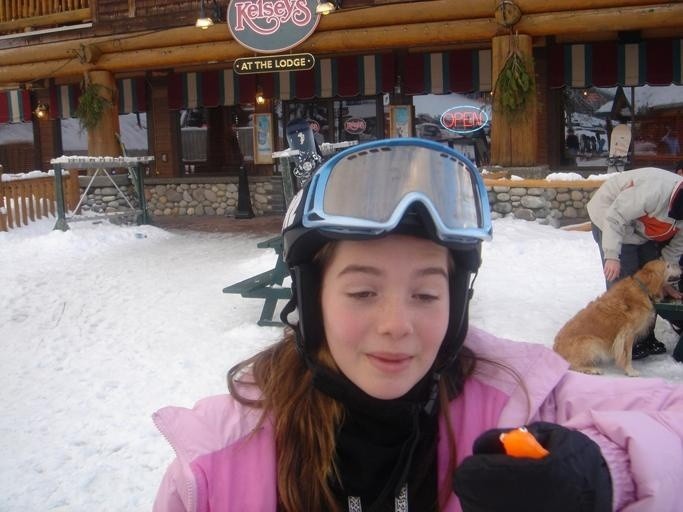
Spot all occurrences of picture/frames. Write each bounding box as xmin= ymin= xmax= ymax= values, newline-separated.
xmin=336 ymin=92 xmax=385 ymax=149
xmin=282 ymin=97 xmax=335 ymax=149
xmin=254 ymin=113 xmax=276 ymax=165
xmin=389 ymin=105 xmax=413 ymax=139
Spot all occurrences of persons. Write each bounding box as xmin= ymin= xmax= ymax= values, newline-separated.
xmin=585 ymin=166 xmax=683 ymax=362
xmin=139 ymin=136 xmax=683 ymax=511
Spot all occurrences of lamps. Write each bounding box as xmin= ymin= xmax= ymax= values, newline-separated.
xmin=196 ymin=0 xmax=222 ymax=30
xmin=315 ymin=0 xmax=342 ymax=16
xmin=32 ymin=103 xmax=49 ymax=119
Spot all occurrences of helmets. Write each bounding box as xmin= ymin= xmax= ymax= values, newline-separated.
xmin=280 ymin=137 xmax=484 ymax=274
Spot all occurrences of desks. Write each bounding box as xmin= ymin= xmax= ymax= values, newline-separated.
xmin=257 ymin=234 xmax=296 ymax=328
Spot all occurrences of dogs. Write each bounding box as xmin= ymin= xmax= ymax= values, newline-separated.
xmin=553 ymin=260 xmax=682 ymax=377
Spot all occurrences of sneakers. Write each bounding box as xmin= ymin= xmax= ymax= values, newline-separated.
xmin=631 ymin=323 xmax=667 ymax=360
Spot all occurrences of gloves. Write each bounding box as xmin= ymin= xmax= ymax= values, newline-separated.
xmin=450 ymin=419 xmax=615 ymax=512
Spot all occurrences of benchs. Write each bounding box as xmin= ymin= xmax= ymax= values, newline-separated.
xmin=653 ymin=282 xmax=683 ymax=313
xmin=222 ymin=261 xmax=290 ymax=327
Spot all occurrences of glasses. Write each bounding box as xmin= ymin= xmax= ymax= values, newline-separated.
xmin=301 ymin=137 xmax=493 ymax=246
